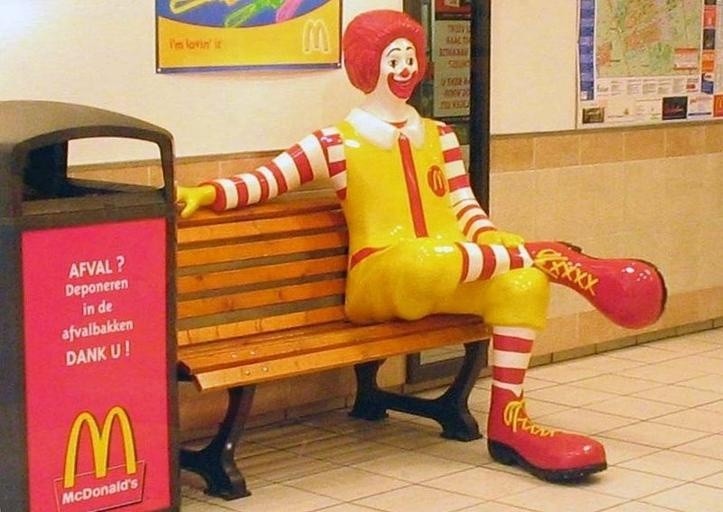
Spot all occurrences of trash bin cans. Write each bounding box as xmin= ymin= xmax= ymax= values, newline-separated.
xmin=0 ymin=100 xmax=181 ymax=512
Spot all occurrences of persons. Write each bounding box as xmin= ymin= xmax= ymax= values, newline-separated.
xmin=169 ymin=9 xmax=668 ymax=485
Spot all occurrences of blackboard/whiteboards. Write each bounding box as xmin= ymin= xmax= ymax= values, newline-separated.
xmin=470 ymin=0 xmax=723 ymax=135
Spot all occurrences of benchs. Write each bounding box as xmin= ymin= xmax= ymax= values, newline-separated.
xmin=170 ymin=179 xmax=493 ymax=504
